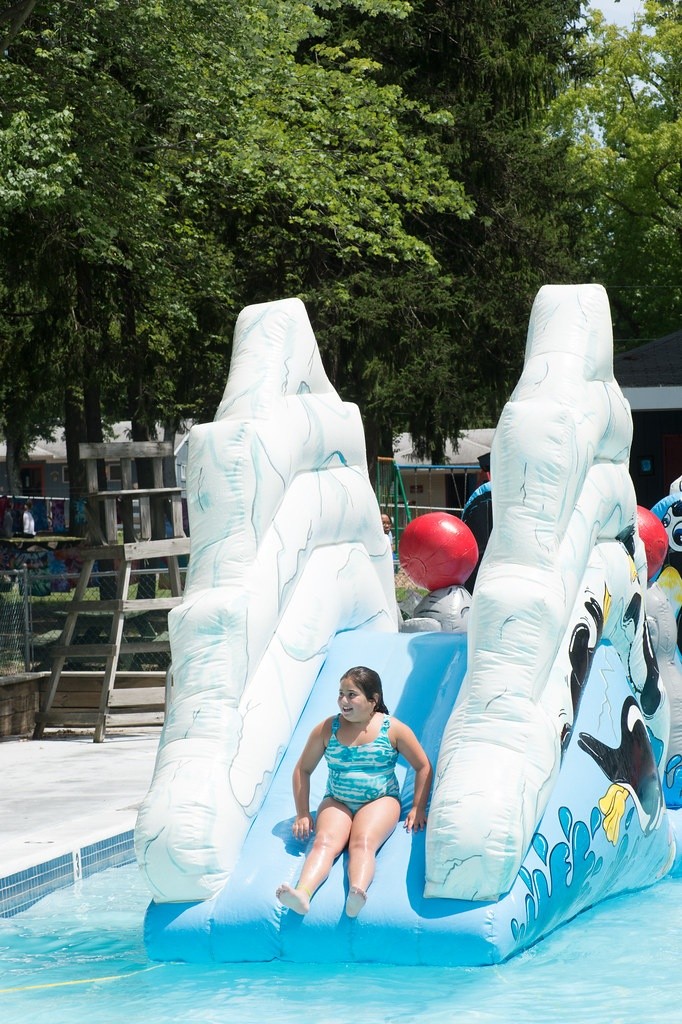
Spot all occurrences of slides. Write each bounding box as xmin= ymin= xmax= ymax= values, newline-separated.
xmin=128 ymin=626 xmax=682 ymax=968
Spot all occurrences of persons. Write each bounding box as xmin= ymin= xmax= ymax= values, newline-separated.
xmin=274 ymin=666 xmax=433 ymax=918
xmin=21 ymin=499 xmax=36 ymax=549
xmin=381 ymin=513 xmax=393 ymax=552
xmin=2 ymin=498 xmax=14 ymax=538
